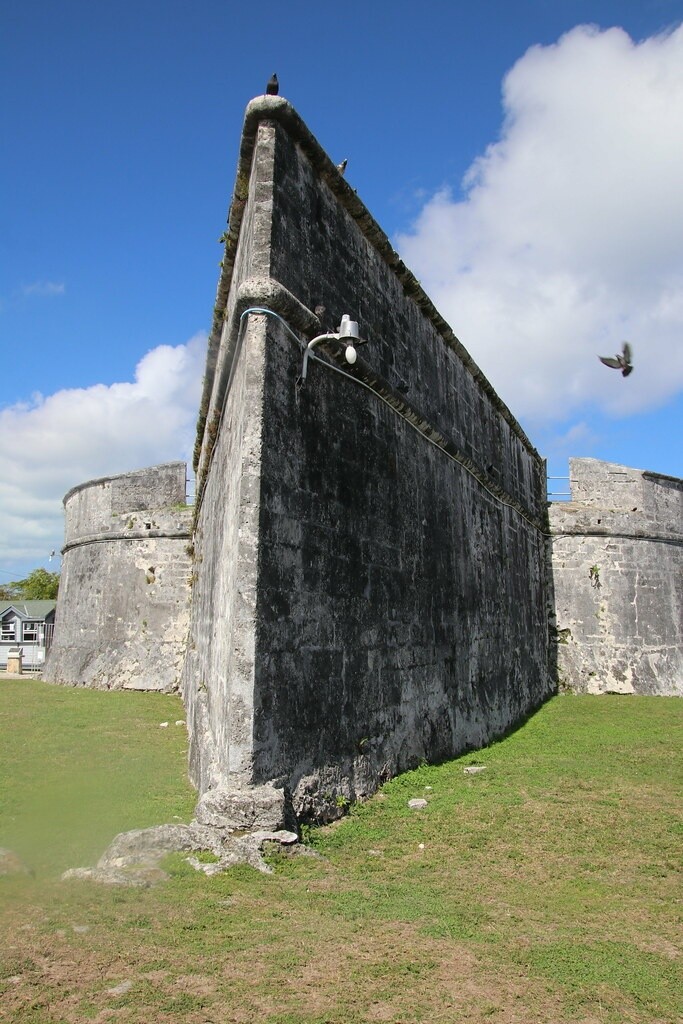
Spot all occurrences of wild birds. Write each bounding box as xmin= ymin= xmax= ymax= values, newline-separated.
xmin=336 ymin=159 xmax=347 ymax=175
xmin=266 ymin=73 xmax=278 ymax=95
xmin=354 ymin=188 xmax=358 ymax=193
xmin=595 ymin=343 xmax=633 ymax=378
